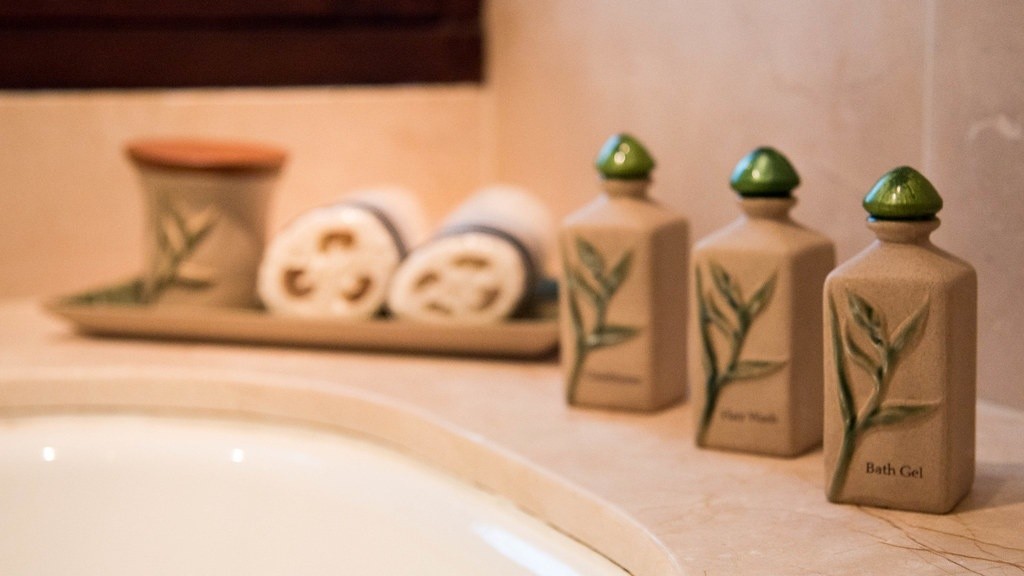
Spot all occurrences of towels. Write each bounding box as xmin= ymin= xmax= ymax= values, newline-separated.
xmin=257 ymin=185 xmax=561 ymax=327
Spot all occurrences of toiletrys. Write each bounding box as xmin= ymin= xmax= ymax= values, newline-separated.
xmin=818 ymin=165 xmax=981 ymax=515
xmin=690 ymin=144 xmax=833 ymax=458
xmin=559 ymin=133 xmax=689 ymax=413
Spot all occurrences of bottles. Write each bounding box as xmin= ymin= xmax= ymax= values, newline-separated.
xmin=689 ymin=150 xmax=840 ymax=458
xmin=560 ymin=136 xmax=689 ymax=411
xmin=824 ymin=165 xmax=979 ymax=512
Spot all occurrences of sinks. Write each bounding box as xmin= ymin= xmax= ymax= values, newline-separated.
xmin=3 ymin=375 xmax=690 ymax=576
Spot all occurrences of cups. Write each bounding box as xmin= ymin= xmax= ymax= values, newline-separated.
xmin=123 ymin=140 xmax=287 ymax=308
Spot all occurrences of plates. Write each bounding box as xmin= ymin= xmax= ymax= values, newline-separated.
xmin=45 ymin=264 xmax=562 ymax=359
